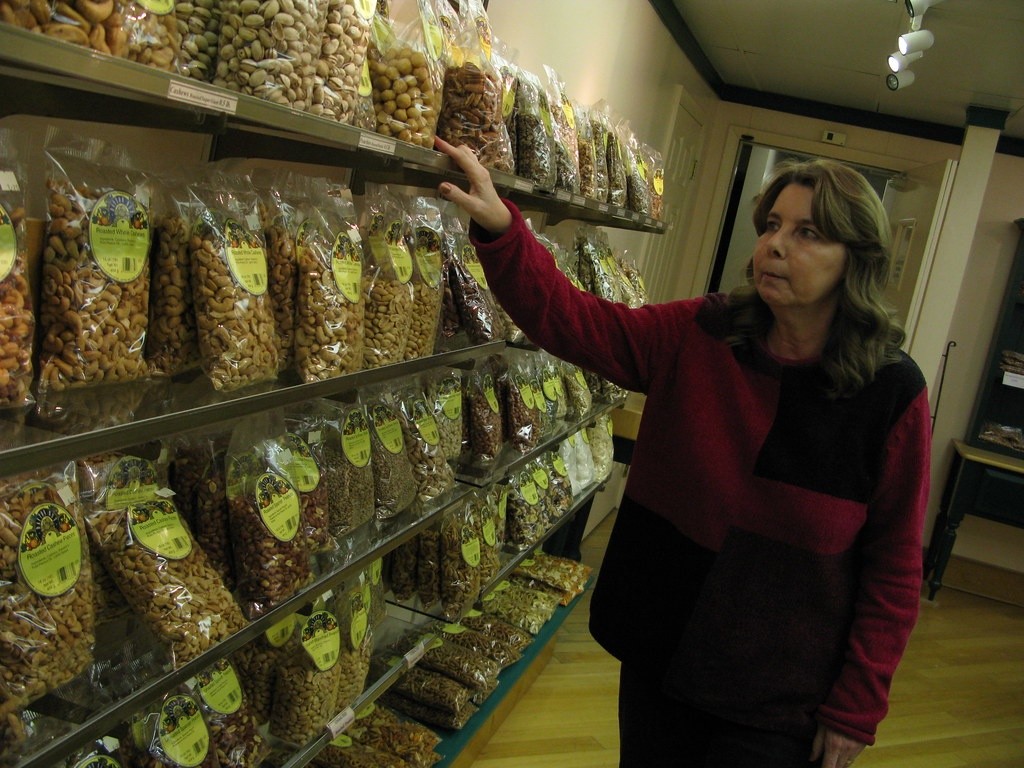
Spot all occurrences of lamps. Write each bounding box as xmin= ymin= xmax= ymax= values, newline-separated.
xmin=886 ymin=0 xmax=940 ymax=91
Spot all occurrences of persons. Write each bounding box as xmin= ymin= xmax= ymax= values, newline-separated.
xmin=430 ymin=137 xmax=931 ymax=768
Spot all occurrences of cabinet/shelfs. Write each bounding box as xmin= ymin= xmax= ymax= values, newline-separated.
xmin=923 ymin=219 xmax=1024 ymax=457
xmin=0 ymin=22 xmax=674 ymax=768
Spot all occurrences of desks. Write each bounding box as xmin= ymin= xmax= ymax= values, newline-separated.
xmin=922 ymin=439 xmax=1024 ymax=601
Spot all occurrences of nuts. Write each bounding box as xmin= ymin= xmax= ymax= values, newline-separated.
xmin=0 ymin=0 xmax=543 ymax=768
